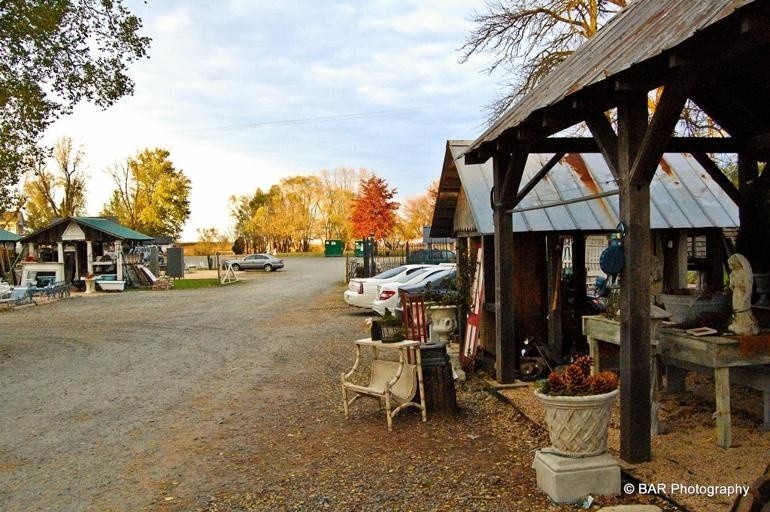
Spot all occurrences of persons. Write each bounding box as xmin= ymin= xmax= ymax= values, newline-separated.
xmin=647 ymin=243 xmax=671 ymax=323
xmin=727 ymin=253 xmax=759 ymax=329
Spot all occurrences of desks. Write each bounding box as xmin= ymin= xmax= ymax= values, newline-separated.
xmin=341 ymin=337 xmax=427 ymax=432
xmin=580 ymin=314 xmax=770 ymax=448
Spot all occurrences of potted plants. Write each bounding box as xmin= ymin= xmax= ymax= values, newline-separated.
xmin=535 ymin=354 xmax=619 ymax=459
xmin=379 ymin=308 xmax=402 ymax=343
xmin=406 ymin=243 xmax=477 ymax=349
xmin=659 ymin=286 xmax=725 ymax=330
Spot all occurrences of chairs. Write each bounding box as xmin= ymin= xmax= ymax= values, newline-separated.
xmin=0 ymin=284 xmax=29 ymax=310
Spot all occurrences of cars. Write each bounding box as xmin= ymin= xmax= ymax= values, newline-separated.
xmin=144 ymin=257 xmax=190 ymax=272
xmin=228 ymin=254 xmax=284 ymax=272
xmin=345 ymin=249 xmax=456 ymax=318
